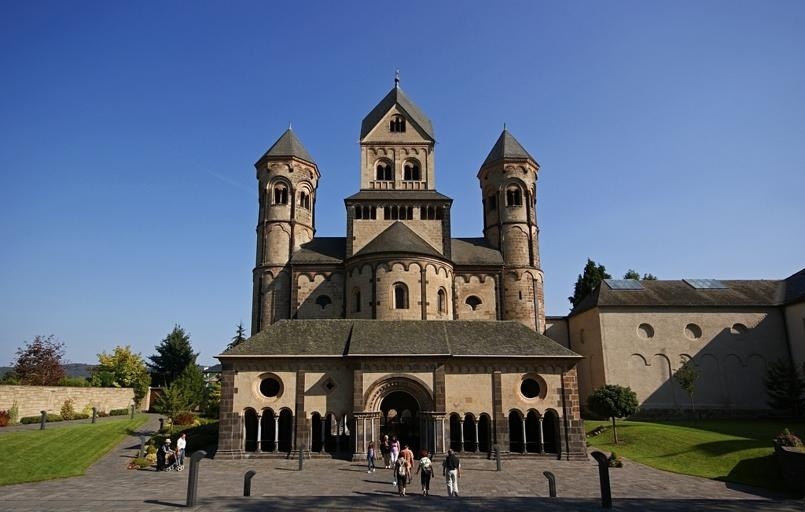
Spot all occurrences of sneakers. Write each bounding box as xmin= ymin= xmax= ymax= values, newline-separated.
xmin=367 ymin=465 xmax=392 ymax=473
xmin=400 ymin=489 xmax=458 ymax=497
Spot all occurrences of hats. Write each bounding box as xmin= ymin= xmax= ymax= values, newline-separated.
xmin=164 ymin=439 xmax=171 ymax=442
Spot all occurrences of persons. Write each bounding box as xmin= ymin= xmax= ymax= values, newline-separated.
xmin=155 ymin=439 xmax=174 ymax=472
xmin=393 ymin=453 xmax=412 ymax=497
xmin=412 ymin=449 xmax=435 ymax=497
xmin=367 ymin=441 xmax=377 ymax=473
xmin=390 ymin=436 xmax=400 ymax=469
xmin=442 ymin=449 xmax=461 ymax=497
xmin=399 ymin=445 xmax=415 ymax=484
xmin=380 ymin=434 xmax=391 ymax=469
xmin=176 ymin=432 xmax=187 ymax=466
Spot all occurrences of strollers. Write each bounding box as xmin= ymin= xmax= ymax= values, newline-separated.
xmin=164 ymin=449 xmax=185 ymax=472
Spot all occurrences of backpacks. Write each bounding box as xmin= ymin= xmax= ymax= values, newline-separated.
xmin=421 ymin=459 xmax=432 ymax=475
xmin=397 ymin=459 xmax=406 ymax=476
